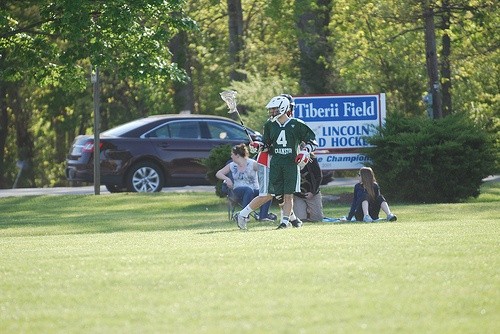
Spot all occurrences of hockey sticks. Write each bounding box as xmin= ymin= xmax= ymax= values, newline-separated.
xmin=218 ymin=90 xmax=253 ymax=143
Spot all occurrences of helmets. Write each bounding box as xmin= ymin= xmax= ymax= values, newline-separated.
xmin=265 ymin=96 xmax=290 ymax=122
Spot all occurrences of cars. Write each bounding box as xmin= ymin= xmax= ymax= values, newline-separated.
xmin=66 ymin=114 xmax=262 ymax=192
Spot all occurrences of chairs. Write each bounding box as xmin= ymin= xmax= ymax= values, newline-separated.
xmin=221 ymin=160 xmax=260 ymax=221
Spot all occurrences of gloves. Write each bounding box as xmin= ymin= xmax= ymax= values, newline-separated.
xmin=249 ymin=141 xmax=268 ymax=153
xmin=295 ymin=148 xmax=310 ymax=170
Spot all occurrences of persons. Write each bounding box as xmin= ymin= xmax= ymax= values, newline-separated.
xmin=341 ymin=167 xmax=397 ymax=223
xmin=216 ymin=94 xmax=322 ymax=231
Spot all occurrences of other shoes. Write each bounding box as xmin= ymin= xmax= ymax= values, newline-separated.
xmin=233 ymin=211 xmax=247 ymax=230
xmin=387 ymin=213 xmax=397 ymax=222
xmin=291 ymin=218 xmax=302 ymax=228
xmin=363 ymin=215 xmax=374 ymax=223
xmin=277 ymin=221 xmax=292 ymax=230
